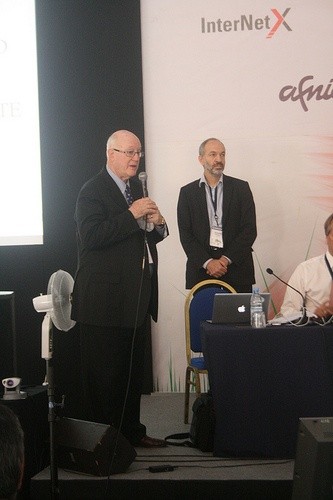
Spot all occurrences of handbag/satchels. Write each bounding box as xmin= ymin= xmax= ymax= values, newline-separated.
xmin=165 ymin=392 xmax=214 ymax=451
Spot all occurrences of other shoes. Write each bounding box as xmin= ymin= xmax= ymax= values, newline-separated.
xmin=135 ymin=434 xmax=167 ymax=447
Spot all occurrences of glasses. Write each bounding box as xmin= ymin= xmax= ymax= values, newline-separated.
xmin=113 ymin=148 xmax=142 ymax=158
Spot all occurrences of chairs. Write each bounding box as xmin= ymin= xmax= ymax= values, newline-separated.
xmin=184 ymin=279 xmax=237 ymax=424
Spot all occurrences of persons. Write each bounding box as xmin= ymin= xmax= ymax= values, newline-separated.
xmin=70 ymin=130 xmax=169 ymax=447
xmin=281 ymin=214 xmax=333 ymax=318
xmin=177 ymin=139 xmax=257 ymax=293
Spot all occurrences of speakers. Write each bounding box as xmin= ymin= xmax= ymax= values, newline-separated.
xmin=293 ymin=417 xmax=333 ymax=500
xmin=54 ymin=415 xmax=137 ymax=476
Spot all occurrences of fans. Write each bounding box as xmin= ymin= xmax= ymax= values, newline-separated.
xmin=32 ymin=268 xmax=77 ymax=386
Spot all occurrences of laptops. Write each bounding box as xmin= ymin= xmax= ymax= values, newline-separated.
xmin=206 ymin=292 xmax=272 ymax=323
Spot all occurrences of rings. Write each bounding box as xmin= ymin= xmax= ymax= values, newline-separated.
xmin=151 ymin=214 xmax=152 ymax=218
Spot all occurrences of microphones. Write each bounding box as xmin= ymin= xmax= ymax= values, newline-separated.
xmin=266 ymin=268 xmax=306 ymax=318
xmin=138 ymin=172 xmax=148 ymax=198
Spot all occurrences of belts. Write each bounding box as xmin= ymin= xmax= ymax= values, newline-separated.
xmin=210 ymin=245 xmax=225 ymax=251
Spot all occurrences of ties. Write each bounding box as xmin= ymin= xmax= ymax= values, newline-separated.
xmin=124 ymin=183 xmax=134 ymax=207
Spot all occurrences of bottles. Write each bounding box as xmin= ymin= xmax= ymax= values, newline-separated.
xmin=249 ymin=284 xmax=263 ymax=325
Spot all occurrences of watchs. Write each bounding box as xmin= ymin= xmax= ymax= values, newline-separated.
xmin=157 ymin=216 xmax=166 ymax=226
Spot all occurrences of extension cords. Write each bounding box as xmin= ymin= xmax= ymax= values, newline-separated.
xmin=149 ymin=465 xmax=174 ymax=472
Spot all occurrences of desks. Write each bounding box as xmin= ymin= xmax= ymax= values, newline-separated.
xmin=199 ymin=316 xmax=333 ymax=459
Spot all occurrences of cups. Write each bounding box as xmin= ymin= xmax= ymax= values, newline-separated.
xmin=252 ymin=311 xmax=266 ymax=328
